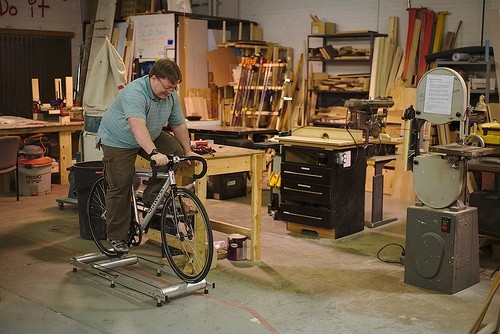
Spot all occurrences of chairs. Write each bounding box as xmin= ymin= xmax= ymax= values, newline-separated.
xmin=0 ymin=135 xmax=21 ymax=201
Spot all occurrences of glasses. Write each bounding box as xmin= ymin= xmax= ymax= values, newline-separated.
xmin=157 ymin=78 xmax=177 ymax=94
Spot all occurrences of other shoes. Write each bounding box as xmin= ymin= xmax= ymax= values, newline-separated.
xmin=107 ymin=236 xmax=129 ymax=253
xmin=148 ymin=201 xmax=163 ymax=209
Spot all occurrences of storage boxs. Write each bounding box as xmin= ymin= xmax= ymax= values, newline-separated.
xmin=311 ymin=20 xmax=337 ymax=34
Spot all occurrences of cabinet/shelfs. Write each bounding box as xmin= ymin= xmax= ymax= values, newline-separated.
xmin=435 ymin=58 xmax=498 ymax=96
xmin=274 ymin=135 xmax=368 ymax=241
xmin=304 ymin=31 xmax=389 ymax=130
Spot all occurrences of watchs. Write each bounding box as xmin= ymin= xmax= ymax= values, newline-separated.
xmin=150 ymin=149 xmax=158 ymax=157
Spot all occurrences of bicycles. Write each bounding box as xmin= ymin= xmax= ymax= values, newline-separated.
xmin=85 ymin=155 xmax=214 ymax=284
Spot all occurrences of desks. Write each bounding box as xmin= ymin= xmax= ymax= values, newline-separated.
xmin=136 ymin=144 xmax=265 ymax=274
xmin=188 ymin=125 xmax=276 ymax=138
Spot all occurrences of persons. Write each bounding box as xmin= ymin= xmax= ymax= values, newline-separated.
xmin=98 ymin=59 xmax=201 ymax=253
